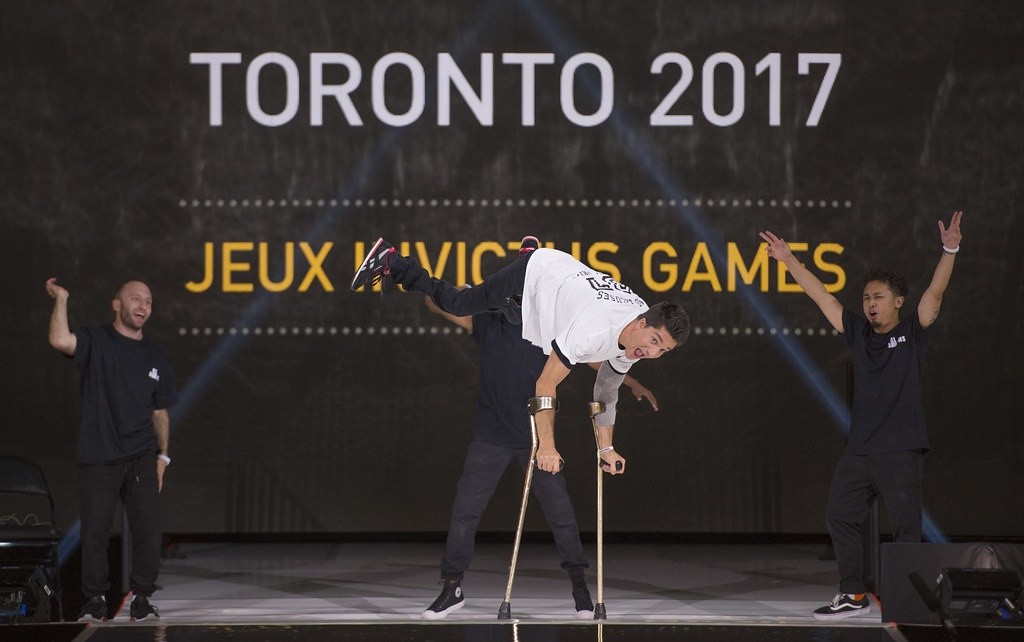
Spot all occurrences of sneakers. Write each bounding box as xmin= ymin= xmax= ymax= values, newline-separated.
xmin=571 ymin=586 xmax=595 ymax=620
xmin=129 ymin=601 xmax=159 ymax=622
xmin=421 ymin=580 xmax=466 ymax=621
xmin=350 ymin=237 xmax=396 ymax=292
xmin=78 ymin=599 xmax=108 ymax=623
xmin=518 ymin=236 xmax=539 ymax=253
xmin=811 ymin=594 xmax=871 ymax=621
xmin=870 ymin=592 xmax=882 ymax=608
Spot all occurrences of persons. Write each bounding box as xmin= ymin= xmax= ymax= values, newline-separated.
xmin=759 ymin=210 xmax=963 ymax=621
xmin=350 ymin=236 xmax=690 ymax=474
xmin=422 ymin=293 xmax=659 ymax=619
xmin=47 ymin=277 xmax=177 ymax=622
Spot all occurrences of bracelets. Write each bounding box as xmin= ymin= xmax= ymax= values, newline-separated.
xmin=598 ymin=445 xmax=615 ymax=454
xmin=158 ymin=454 xmax=171 ymax=466
xmin=942 ymin=244 xmax=961 ymax=253
